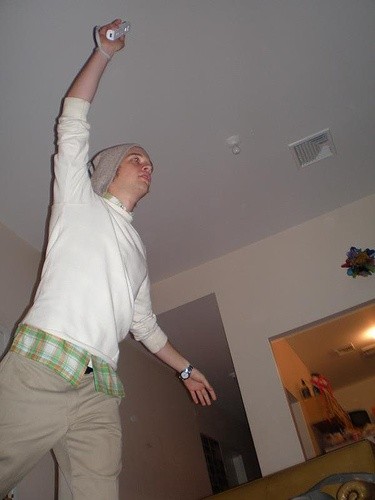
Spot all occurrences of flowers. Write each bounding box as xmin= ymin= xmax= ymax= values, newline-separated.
xmin=341 ymin=247 xmax=375 ymax=279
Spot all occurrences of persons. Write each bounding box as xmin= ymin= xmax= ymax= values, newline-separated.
xmin=0 ymin=18 xmax=216 ymax=500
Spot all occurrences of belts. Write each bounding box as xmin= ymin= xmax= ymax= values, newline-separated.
xmin=84 ymin=367 xmax=92 ymax=375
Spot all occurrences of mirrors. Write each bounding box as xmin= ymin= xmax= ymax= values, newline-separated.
xmin=268 ymin=299 xmax=375 ymax=459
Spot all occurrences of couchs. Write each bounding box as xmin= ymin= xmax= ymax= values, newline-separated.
xmin=201 ymin=439 xmax=375 ymax=500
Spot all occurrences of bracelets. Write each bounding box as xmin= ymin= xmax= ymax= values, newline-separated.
xmin=176 ymin=365 xmax=195 ymax=382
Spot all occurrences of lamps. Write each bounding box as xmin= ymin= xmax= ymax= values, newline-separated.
xmin=226 ymin=135 xmax=241 ymax=155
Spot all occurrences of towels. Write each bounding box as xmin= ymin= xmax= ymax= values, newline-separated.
xmin=88 ymin=143 xmax=140 ymax=196
